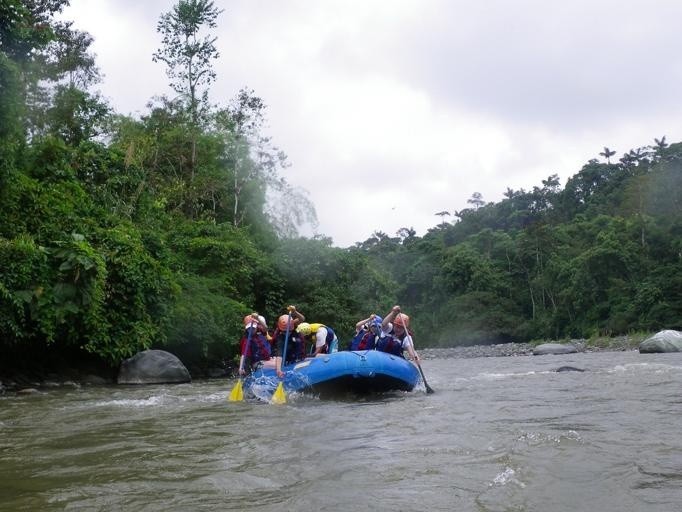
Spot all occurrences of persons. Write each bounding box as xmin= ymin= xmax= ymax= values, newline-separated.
xmin=244 ymin=313 xmax=274 ymax=341
xmin=352 ymin=313 xmax=382 ymax=350
xmin=239 ymin=323 xmax=282 ymax=375
xmin=295 ymin=323 xmax=338 ymax=355
xmin=375 ymin=305 xmax=421 ymax=366
xmin=271 ymin=304 xmax=305 ymax=376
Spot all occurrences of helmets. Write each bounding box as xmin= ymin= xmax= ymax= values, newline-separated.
xmin=367 ymin=314 xmax=383 ymax=329
xmin=295 ymin=321 xmax=312 ymax=338
xmin=393 ymin=313 xmax=409 ymax=327
xmin=278 ymin=314 xmax=294 ymax=332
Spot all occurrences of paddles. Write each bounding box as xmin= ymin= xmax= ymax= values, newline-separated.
xmin=272 ymin=311 xmax=292 ymax=404
xmin=229 ymin=316 xmax=255 ymax=402
xmin=398 ymin=308 xmax=434 ymax=395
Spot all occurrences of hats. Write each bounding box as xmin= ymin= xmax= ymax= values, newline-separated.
xmin=243 ymin=315 xmax=259 ymax=329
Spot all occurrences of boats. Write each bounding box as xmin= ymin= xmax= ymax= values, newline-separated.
xmin=237 ymin=348 xmax=421 ymax=404
